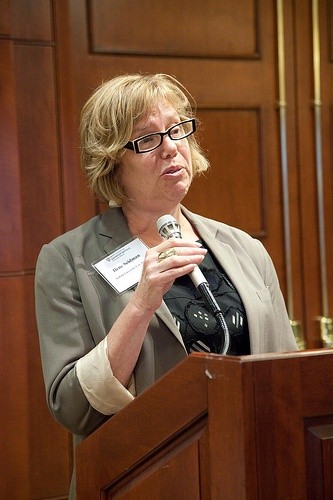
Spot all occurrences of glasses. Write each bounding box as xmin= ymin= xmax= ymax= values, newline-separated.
xmin=123 ymin=115 xmax=197 ymax=154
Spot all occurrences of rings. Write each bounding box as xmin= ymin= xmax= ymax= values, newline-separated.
xmin=158 ymin=247 xmax=178 ymax=259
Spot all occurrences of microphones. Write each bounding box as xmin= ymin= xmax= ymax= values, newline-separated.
xmin=156 ymin=214 xmax=229 ymax=355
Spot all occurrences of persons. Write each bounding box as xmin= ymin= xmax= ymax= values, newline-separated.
xmin=36 ymin=73 xmax=300 ymax=500
